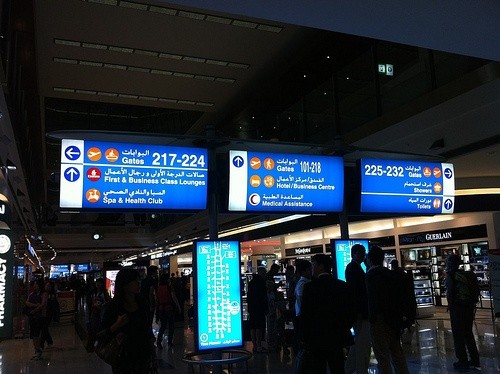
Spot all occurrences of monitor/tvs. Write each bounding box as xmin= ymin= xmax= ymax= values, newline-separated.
xmin=330 ymin=238 xmax=370 ymax=282
xmin=193 ymin=239 xmax=244 ymax=352
xmin=60 ymin=138 xmax=209 ymax=213
xmin=229 ymin=149 xmax=344 ymax=212
xmin=358 ymin=157 xmax=454 ymax=215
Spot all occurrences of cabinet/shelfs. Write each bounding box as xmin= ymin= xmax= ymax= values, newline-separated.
xmin=400 ymin=241 xmax=490 ymax=305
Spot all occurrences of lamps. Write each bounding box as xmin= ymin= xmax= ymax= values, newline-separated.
xmin=429 ymin=138 xmax=444 ymax=151
xmin=6 ymin=159 xmax=17 ymax=171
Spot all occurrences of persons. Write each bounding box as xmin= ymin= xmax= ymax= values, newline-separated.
xmin=25 ymin=265 xmax=181 ymax=374
xmin=445 ymin=255 xmax=482 ymax=370
xmin=247 ymin=244 xmax=416 ymax=374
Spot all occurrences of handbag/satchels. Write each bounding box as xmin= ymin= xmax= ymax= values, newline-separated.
xmin=96 ymin=307 xmax=133 ymax=370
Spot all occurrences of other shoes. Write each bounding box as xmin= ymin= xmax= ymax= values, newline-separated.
xmin=454 ymin=361 xmax=481 ymax=369
xmin=31 ymin=352 xmax=43 ymax=361
xmin=47 ymin=345 xmax=55 ymax=350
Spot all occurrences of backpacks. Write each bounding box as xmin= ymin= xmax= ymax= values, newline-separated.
xmin=369 ymin=268 xmax=421 ymax=339
xmin=447 ymin=270 xmax=482 ymax=307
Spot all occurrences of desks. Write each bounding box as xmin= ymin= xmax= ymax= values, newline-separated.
xmin=414 ymin=313 xmax=450 ymax=350
